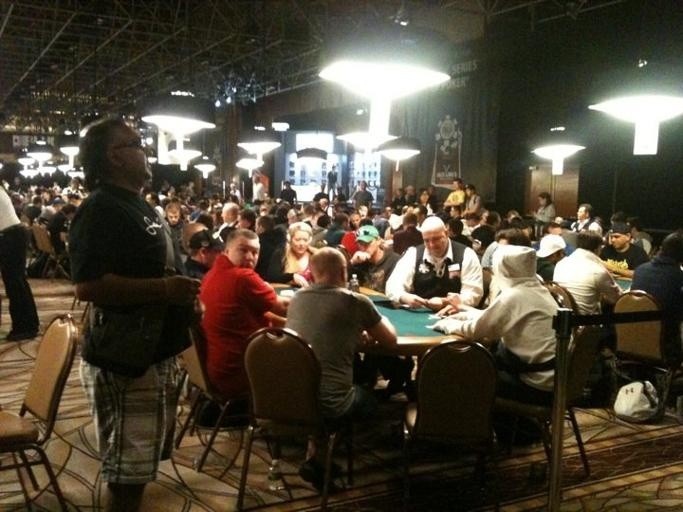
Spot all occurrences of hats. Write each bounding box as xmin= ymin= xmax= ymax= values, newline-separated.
xmin=353 ymin=224 xmax=380 ymax=244
xmin=188 ymin=228 xmax=226 ymax=252
xmin=608 ymin=222 xmax=631 ymax=234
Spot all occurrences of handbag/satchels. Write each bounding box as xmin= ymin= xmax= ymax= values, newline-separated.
xmin=612 ymin=379 xmax=661 ymax=424
xmin=77 ymin=305 xmax=160 ymax=380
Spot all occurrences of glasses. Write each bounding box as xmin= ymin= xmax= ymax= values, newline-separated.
xmin=110 ymin=134 xmax=147 ymax=151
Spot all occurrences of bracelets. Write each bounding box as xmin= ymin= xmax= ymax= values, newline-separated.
xmin=162 ymin=278 xmax=170 ymax=298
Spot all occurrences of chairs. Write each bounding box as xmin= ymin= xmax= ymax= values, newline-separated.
xmin=1 ymin=312 xmax=81 ymax=510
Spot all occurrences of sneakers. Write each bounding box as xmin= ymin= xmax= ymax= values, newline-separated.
xmin=298 ymin=455 xmax=345 ymax=495
xmin=5 ymin=324 xmax=40 ymax=342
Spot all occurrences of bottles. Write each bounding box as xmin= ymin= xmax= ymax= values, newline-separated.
xmin=348 ymin=274 xmax=359 ymax=293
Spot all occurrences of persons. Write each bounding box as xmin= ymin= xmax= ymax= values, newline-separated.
xmin=0 ymin=175 xmax=89 ymax=279
xmin=0 ymin=186 xmax=41 ymax=341
xmin=139 ymin=172 xmax=683 ymax=496
xmin=64 ymin=115 xmax=208 ymax=512
xmin=327 ymin=165 xmax=338 ymax=201
